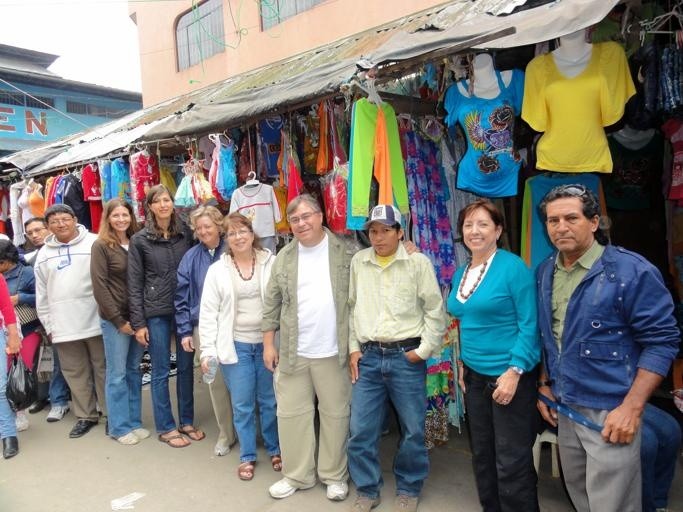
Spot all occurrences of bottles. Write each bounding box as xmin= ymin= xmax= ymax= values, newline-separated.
xmin=201 ymin=353 xmax=218 ymax=387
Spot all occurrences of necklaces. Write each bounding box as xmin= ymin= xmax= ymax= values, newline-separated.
xmin=231 ymin=253 xmax=256 ymax=281
xmin=458 ymin=258 xmax=487 ymax=300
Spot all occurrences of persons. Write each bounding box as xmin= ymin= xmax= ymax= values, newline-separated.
xmin=90 ymin=197 xmax=149 ymax=445
xmin=23 ymin=217 xmax=70 ymax=422
xmin=520 ymin=22 xmax=632 ymax=172
xmin=445 ymin=52 xmax=526 ymax=197
xmin=33 ymin=205 xmax=108 ymax=439
xmin=199 ymin=213 xmax=283 ymax=481
xmin=0 ymin=271 xmax=38 ymax=458
xmin=125 ymin=183 xmax=206 ymax=449
xmin=174 ymin=205 xmax=238 ymax=458
xmin=346 ymin=205 xmax=446 ymax=512
xmin=534 ymin=183 xmax=678 ymax=512
xmin=446 ymin=197 xmax=544 ymax=511
xmin=263 ymin=195 xmax=422 ymax=503
xmin=1 ymin=238 xmax=36 ymax=409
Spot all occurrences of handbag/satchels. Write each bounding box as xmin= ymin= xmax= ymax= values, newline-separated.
xmin=37 ymin=339 xmax=54 ymax=371
xmin=15 ymin=267 xmax=37 ymax=325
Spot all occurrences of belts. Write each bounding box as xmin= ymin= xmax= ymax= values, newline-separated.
xmin=370 ymin=340 xmax=420 ymax=348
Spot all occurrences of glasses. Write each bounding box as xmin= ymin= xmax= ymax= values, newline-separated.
xmin=290 ymin=211 xmax=318 ymax=224
xmin=545 ymin=184 xmax=587 ymax=200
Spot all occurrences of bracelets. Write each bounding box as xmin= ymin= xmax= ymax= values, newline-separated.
xmin=509 ymin=365 xmax=523 ymax=375
xmin=535 ymin=380 xmax=551 ymax=388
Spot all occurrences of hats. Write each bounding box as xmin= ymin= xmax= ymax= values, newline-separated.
xmin=364 ymin=205 xmax=402 ymax=229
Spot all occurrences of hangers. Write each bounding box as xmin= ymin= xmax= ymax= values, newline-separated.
xmin=363 ymin=78 xmax=383 ymax=103
xmin=135 ymin=141 xmax=147 ymax=152
xmin=207 ymin=128 xmax=238 ymax=152
xmin=60 ymin=152 xmax=122 ymax=175
xmin=242 ymin=170 xmax=259 ymax=189
xmin=298 ymin=104 xmax=317 ymax=126
xmin=331 ymin=154 xmax=340 ymax=170
xmin=627 ymin=4 xmax=682 ymax=41
